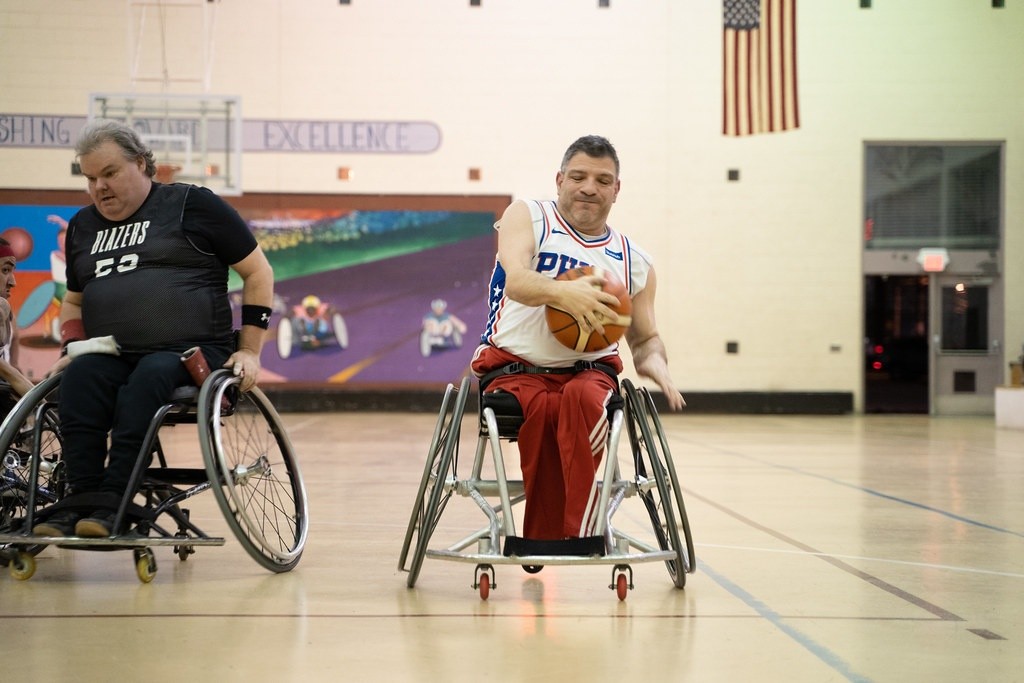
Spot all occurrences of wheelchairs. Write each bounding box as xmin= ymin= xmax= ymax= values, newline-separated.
xmin=0 ymin=329 xmax=308 ymax=584
xmin=398 ymin=377 xmax=696 ymax=600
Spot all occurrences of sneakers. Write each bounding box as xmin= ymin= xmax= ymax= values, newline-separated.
xmin=74 ymin=508 xmax=116 ymax=537
xmin=33 ymin=510 xmax=80 ymax=537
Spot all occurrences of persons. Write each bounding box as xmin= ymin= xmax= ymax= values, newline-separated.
xmin=30 ymin=119 xmax=275 ymax=537
xmin=421 ymin=298 xmax=466 ymax=343
xmin=0 ymin=239 xmax=53 ymax=469
xmin=471 ymin=135 xmax=688 ymax=559
xmin=291 ymin=294 xmax=332 ymax=340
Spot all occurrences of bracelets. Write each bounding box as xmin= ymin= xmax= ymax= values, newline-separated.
xmin=241 ymin=303 xmax=272 ymax=330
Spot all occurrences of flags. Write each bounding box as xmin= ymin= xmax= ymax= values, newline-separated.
xmin=719 ymin=0 xmax=801 ymax=138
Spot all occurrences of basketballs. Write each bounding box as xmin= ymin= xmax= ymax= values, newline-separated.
xmin=2 ymin=226 xmax=34 ymax=261
xmin=545 ymin=266 xmax=632 ymax=352
